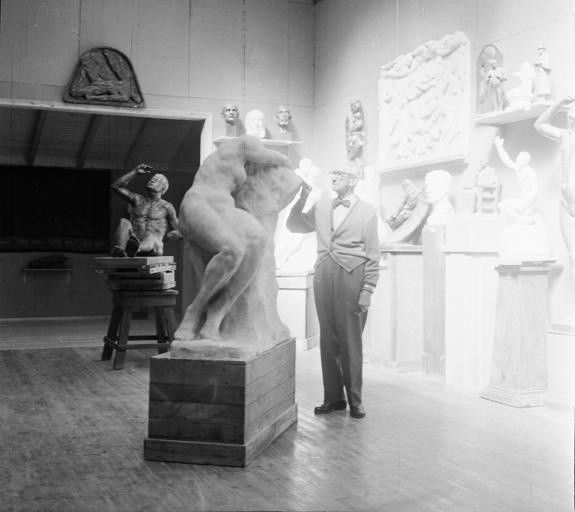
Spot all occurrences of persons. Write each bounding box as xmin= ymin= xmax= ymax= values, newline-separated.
xmin=532 ymin=90 xmax=575 ymax=270
xmin=387 ymin=176 xmax=420 ymax=231
xmin=109 ymin=163 xmax=184 ymax=260
xmin=489 ymin=133 xmax=544 ymax=232
xmin=481 ymin=57 xmax=506 ymax=113
xmin=284 ymin=159 xmax=382 ymax=420
xmin=341 ymin=98 xmax=367 ymax=161
xmin=528 ymin=41 xmax=554 ymax=102
xmin=170 ymin=99 xmax=305 ymax=337
xmin=420 ymin=168 xmax=458 ymax=228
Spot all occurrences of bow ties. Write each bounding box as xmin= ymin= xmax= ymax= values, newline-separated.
xmin=331 ymin=199 xmax=350 ymax=208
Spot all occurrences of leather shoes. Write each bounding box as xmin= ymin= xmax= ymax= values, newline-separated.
xmin=350 ymin=405 xmax=366 ymax=418
xmin=314 ymin=399 xmax=347 ymax=414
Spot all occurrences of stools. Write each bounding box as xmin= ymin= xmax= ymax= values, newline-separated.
xmin=100 ymin=294 xmax=179 ymax=371
